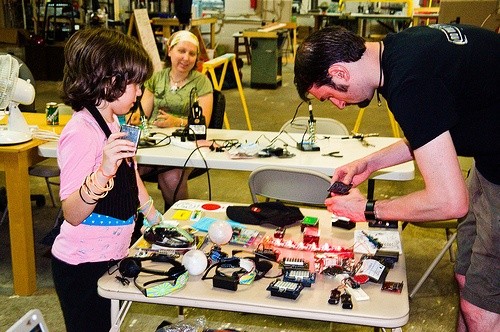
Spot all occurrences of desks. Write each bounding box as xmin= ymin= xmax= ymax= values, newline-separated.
xmin=217 ymin=12 xmax=437 ymax=63
xmin=38 ymin=127 xmax=416 ymax=204
xmin=0 ymin=112 xmax=72 ymax=296
xmin=95 ymin=196 xmax=411 ymax=332
xmin=153 ymin=17 xmax=216 ymax=62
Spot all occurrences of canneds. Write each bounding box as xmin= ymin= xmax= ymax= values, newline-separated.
xmin=45 ymin=102 xmax=59 ymax=125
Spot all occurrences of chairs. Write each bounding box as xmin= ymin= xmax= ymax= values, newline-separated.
xmin=402 ymin=165 xmax=472 ymax=298
xmin=248 ymin=118 xmax=348 ymax=209
xmin=28 ymin=89 xmax=226 ymax=207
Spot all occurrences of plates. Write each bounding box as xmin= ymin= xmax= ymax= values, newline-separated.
xmin=0 ymin=131 xmax=33 ymax=145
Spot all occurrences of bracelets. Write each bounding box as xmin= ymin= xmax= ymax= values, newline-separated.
xmin=138 ymin=196 xmax=164 ymax=226
xmin=79 ymin=164 xmax=116 ymax=205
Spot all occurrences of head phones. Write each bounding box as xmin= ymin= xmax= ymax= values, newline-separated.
xmin=118 ymin=254 xmax=185 ymax=278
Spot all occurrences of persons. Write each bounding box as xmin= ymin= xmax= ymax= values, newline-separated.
xmin=50 ymin=28 xmax=163 ymax=332
xmin=136 ymin=31 xmax=213 ymax=207
xmin=293 ymin=23 xmax=500 ymax=332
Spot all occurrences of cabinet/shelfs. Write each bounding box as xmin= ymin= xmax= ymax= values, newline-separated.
xmin=249 ymin=37 xmax=283 ymax=89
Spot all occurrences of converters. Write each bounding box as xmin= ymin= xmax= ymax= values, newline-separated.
xmin=213 ymin=275 xmax=238 ymax=291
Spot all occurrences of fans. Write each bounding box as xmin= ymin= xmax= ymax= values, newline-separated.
xmin=0 ymin=53 xmax=35 ymax=145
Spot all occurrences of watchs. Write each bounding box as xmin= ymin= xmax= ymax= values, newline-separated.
xmin=364 ymin=198 xmax=377 ymax=221
